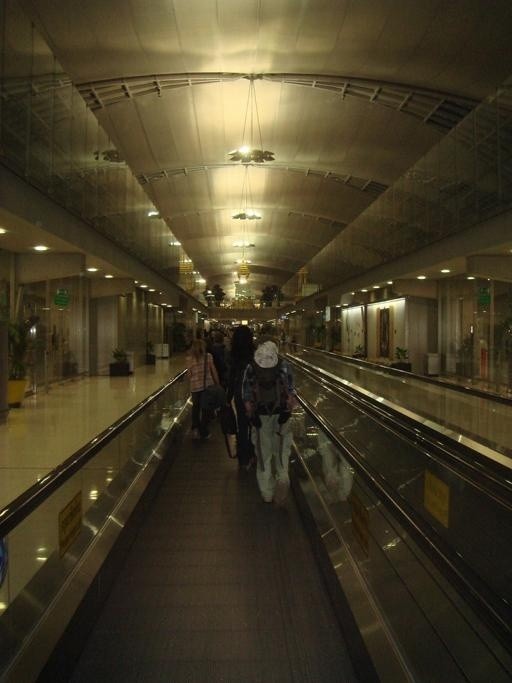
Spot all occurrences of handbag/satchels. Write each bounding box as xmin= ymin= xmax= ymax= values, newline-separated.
xmin=219 ymin=403 xmax=237 ymax=436
xmin=199 ymin=384 xmax=226 ymax=410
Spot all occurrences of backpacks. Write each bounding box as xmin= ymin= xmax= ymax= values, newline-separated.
xmin=245 ymin=361 xmax=289 ymax=414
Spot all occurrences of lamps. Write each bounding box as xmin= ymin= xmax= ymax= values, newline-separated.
xmin=228 ymin=78 xmax=277 ymax=165
xmin=231 ymin=221 xmax=256 ymax=247
xmin=229 ymin=164 xmax=263 ymax=220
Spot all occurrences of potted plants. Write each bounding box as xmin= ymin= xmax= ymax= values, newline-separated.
xmin=7 ymin=315 xmax=42 ymax=408
xmin=387 ymin=347 xmax=412 ymax=378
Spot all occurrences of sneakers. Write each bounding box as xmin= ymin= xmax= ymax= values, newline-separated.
xmin=190 ymin=427 xmax=214 ymax=440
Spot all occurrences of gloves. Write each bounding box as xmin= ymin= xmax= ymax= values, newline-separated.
xmin=276 ymin=414 xmax=291 ymax=424
xmin=249 ymin=413 xmax=261 ymax=428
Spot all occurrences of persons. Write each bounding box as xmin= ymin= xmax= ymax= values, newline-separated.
xmin=184 ymin=339 xmax=220 ymax=441
xmin=241 ymin=340 xmax=298 ymax=502
xmin=202 ymin=328 xmax=233 ymax=387
xmin=224 ymin=326 xmax=257 ymax=466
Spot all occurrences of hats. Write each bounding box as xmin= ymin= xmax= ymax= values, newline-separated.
xmin=253 ymin=341 xmax=279 ymax=369
